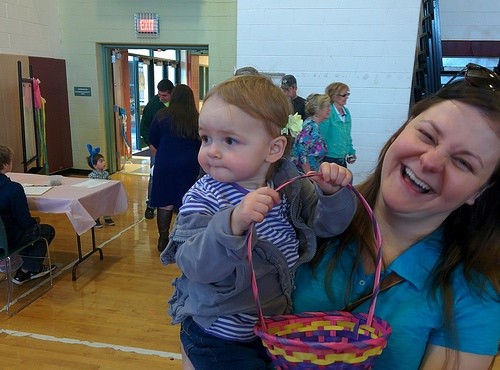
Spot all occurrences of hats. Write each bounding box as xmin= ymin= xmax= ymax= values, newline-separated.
xmin=280 ymin=75 xmax=296 ymax=89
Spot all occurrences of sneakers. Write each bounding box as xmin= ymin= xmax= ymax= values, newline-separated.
xmin=11 ymin=268 xmax=31 ymax=286
xmin=30 ymin=264 xmax=57 ymax=280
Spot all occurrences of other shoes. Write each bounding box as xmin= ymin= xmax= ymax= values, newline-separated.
xmin=105 ymin=219 xmax=115 ymax=226
xmin=95 ymin=220 xmax=103 ymax=228
xmin=145 ymin=207 xmax=154 ymax=219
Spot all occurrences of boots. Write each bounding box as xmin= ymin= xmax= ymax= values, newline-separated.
xmin=157 ymin=209 xmax=173 ymax=252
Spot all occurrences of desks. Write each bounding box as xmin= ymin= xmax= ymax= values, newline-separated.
xmin=5 ymin=173 xmax=129 ymax=281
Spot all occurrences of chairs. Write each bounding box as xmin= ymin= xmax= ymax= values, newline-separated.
xmin=0 ymin=215 xmax=53 ymax=312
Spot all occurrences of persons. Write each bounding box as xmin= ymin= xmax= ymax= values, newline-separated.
xmin=1 ymin=146 xmax=56 ymax=285
xmin=172 ymin=74 xmax=355 ymax=370
xmin=139 ymin=66 xmax=355 ymax=250
xmin=298 ymin=63 xmax=499 ymax=369
xmin=85 ymin=144 xmax=115 ymax=227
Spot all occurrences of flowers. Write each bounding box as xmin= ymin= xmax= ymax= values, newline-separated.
xmin=288 ymin=112 xmax=303 ymax=138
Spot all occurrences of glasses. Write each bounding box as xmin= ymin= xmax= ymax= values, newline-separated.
xmin=443 ymin=63 xmax=500 ymax=91
xmin=338 ymin=93 xmax=350 ymax=97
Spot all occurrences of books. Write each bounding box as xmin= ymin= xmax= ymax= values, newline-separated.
xmin=18 ymin=185 xmax=54 ymax=195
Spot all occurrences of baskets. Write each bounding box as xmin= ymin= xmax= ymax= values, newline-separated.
xmin=248 ymin=173 xmax=393 ymax=370
